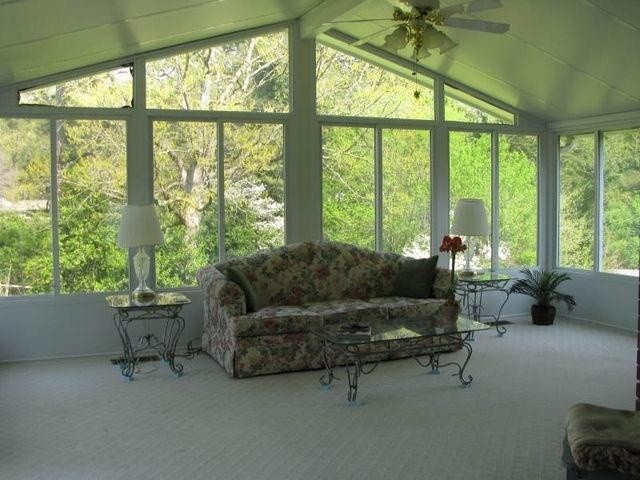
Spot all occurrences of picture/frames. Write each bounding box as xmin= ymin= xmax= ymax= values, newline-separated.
xmin=456 ymin=271 xmax=518 ymax=340
xmin=105 ymin=291 xmax=190 ymax=379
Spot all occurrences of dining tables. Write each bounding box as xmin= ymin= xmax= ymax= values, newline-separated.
xmin=508 ymin=265 xmax=579 ymax=326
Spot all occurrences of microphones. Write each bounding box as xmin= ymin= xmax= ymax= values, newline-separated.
xmin=559 ymin=399 xmax=639 ymax=480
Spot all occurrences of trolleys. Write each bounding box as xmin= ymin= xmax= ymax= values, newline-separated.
xmin=318 ymin=0 xmax=512 ymax=61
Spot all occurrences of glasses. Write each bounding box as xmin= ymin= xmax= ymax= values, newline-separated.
xmin=384 ymin=14 xmax=460 ymax=102
xmin=115 ymin=204 xmax=162 ymax=299
xmin=452 ymin=199 xmax=493 ymax=278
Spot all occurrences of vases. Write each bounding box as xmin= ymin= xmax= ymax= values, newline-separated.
xmin=439 ymin=236 xmax=468 ymax=286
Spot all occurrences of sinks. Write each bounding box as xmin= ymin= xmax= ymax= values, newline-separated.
xmin=192 ymin=240 xmax=463 ymax=381
